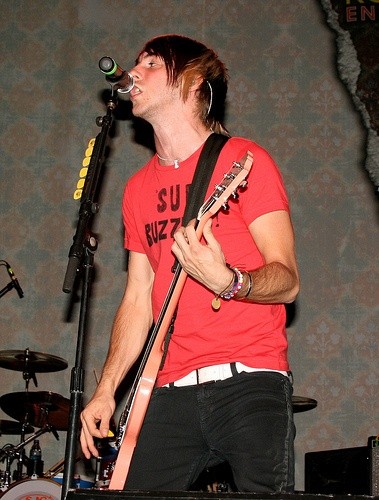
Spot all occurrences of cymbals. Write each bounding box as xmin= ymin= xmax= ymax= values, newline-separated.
xmin=292 ymin=395 xmax=317 ymax=406
xmin=0 ymin=350 xmax=68 ymax=373
xmin=0 ymin=390 xmax=85 ymax=431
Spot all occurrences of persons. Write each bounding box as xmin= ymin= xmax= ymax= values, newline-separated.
xmin=79 ymin=34 xmax=299 ymax=493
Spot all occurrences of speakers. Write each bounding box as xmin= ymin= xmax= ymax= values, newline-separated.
xmin=304 ymin=437 xmax=379 ymax=500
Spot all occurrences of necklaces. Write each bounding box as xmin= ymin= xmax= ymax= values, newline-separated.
xmin=156 ymin=132 xmax=214 ymax=168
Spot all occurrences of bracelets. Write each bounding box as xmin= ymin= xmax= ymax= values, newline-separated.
xmin=212 ymin=263 xmax=253 ymax=310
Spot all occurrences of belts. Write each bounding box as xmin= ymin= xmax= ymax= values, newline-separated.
xmin=162 ymin=361 xmax=289 ymax=386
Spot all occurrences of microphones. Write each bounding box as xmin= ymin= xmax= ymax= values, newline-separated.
xmin=97 ymin=56 xmax=135 ymax=94
xmin=11 ymin=445 xmax=29 ymax=465
xmin=6 ymin=264 xmax=23 ymax=298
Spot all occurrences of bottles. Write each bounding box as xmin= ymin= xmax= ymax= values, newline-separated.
xmin=29 ymin=440 xmax=41 ymax=477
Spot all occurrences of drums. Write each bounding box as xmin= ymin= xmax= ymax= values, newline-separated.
xmin=0 ymin=473 xmax=96 ymax=500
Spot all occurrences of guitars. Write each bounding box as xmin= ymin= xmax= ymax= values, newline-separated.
xmin=107 ymin=149 xmax=253 ymax=491
